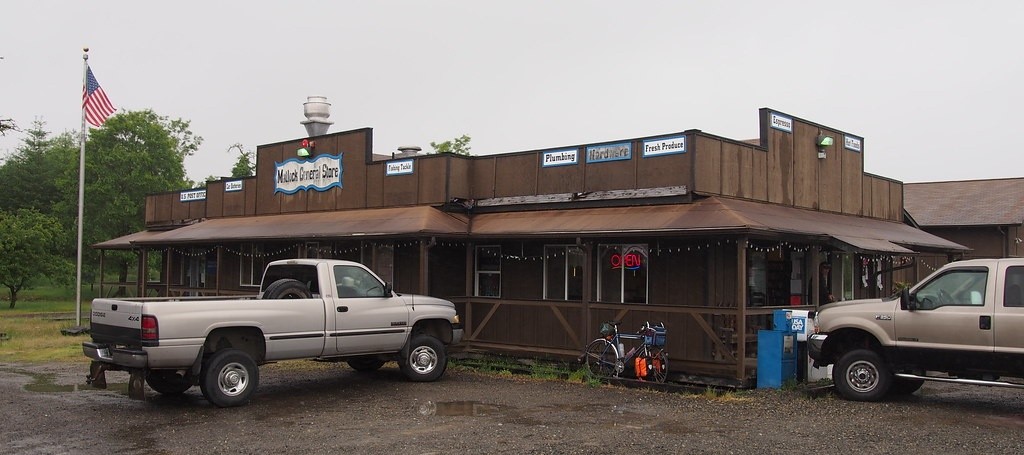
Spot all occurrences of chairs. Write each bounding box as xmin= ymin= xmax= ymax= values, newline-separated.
xmin=1006 ymin=283 xmax=1023 ymax=306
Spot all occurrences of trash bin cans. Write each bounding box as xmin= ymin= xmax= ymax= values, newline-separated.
xmin=773 ymin=309 xmax=834 ymax=382
xmin=756 ymin=331 xmax=798 ymax=391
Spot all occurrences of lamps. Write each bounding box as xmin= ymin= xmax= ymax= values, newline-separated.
xmin=818 ymin=135 xmax=834 ymax=146
xmin=297 ymin=146 xmax=312 ymax=158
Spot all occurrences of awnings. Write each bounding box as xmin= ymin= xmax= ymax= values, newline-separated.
xmin=827 ymin=234 xmax=920 ymax=278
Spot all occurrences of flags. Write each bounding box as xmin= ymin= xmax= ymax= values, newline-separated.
xmin=82 ymin=62 xmax=117 ymax=128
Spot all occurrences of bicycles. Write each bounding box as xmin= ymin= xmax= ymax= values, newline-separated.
xmin=584 ymin=317 xmax=671 ymax=383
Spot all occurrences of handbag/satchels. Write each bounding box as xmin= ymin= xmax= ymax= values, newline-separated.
xmin=635 ymin=357 xmax=661 ymax=377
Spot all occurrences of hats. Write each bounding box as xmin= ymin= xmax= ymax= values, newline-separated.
xmin=820 ymin=262 xmax=832 ymax=270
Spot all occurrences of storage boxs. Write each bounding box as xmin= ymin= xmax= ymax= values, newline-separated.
xmin=757 ymin=309 xmax=833 ymax=389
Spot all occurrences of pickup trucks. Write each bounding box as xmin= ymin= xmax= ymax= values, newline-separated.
xmin=805 ymin=259 xmax=1024 ymax=403
xmin=80 ymin=257 xmax=464 ymax=411
